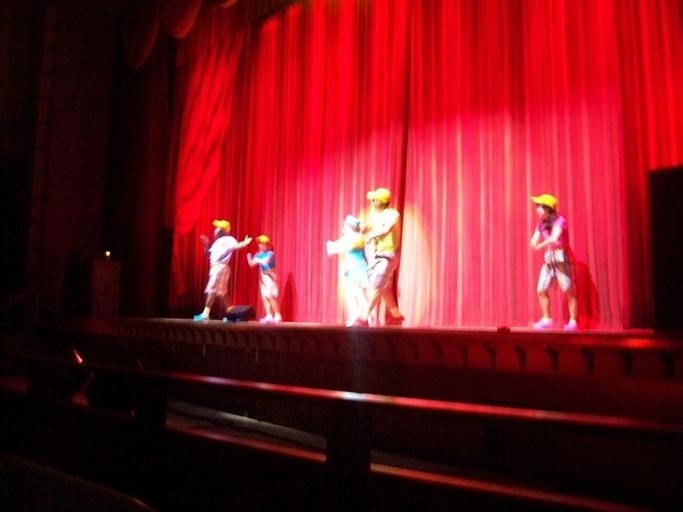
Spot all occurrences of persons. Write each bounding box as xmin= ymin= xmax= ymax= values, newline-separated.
xmin=192 ymin=217 xmax=251 ymax=321
xmin=528 ymin=193 xmax=579 ymax=332
xmin=346 ymin=187 xmax=404 ymax=325
xmin=326 ymin=214 xmax=374 ymax=326
xmin=246 ymin=234 xmax=282 ymax=323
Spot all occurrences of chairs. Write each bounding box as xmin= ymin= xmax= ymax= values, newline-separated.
xmin=0 ymin=454 xmax=152 ymax=512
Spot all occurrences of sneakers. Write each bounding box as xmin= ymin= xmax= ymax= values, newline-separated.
xmin=194 ymin=314 xmax=209 ymax=322
xmin=388 ymin=316 xmax=405 ymax=324
xmin=260 ymin=314 xmax=281 ymax=322
xmin=352 ymin=319 xmax=368 ymax=328
xmin=564 ymin=323 xmax=576 ymax=329
xmin=532 ymin=318 xmax=551 ymax=328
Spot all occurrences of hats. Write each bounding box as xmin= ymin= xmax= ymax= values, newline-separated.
xmin=368 ymin=188 xmax=392 ymax=204
xmin=531 ymin=193 xmax=557 ymax=209
xmin=257 ymin=235 xmax=270 ymax=244
xmin=214 ymin=219 xmax=231 ymax=234
